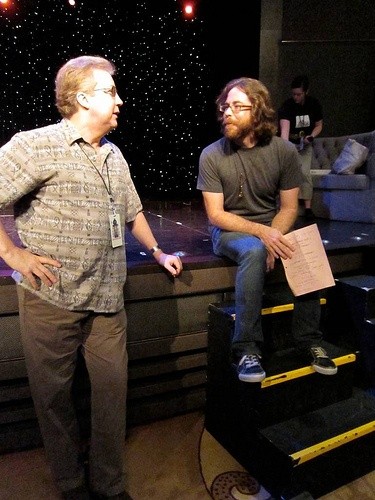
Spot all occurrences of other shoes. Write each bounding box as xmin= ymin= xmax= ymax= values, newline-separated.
xmin=304 ymin=208 xmax=316 ymax=219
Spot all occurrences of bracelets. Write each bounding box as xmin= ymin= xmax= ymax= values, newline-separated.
xmin=149 ymin=245 xmax=161 ymax=253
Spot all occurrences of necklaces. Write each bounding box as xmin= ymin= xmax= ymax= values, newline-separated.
xmin=234 ymin=148 xmax=259 ymax=199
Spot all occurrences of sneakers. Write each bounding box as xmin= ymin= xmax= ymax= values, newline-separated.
xmin=235 ymin=353 xmax=266 ymax=382
xmin=307 ymin=346 xmax=338 ymax=375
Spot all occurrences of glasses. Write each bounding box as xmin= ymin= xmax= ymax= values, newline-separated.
xmin=220 ymin=104 xmax=252 ymax=112
xmin=89 ymin=85 xmax=117 ymax=97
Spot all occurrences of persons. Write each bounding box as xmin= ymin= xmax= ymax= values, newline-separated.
xmin=0 ymin=56 xmax=182 ymax=499
xmin=195 ymin=77 xmax=337 ymax=383
xmin=278 ymin=81 xmax=323 ymax=220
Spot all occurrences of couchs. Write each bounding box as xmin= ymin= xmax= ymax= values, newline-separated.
xmin=312 ymin=131 xmax=375 ymax=223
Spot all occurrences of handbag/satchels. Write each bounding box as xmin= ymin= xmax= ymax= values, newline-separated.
xmin=331 ymin=136 xmax=370 ymax=175
